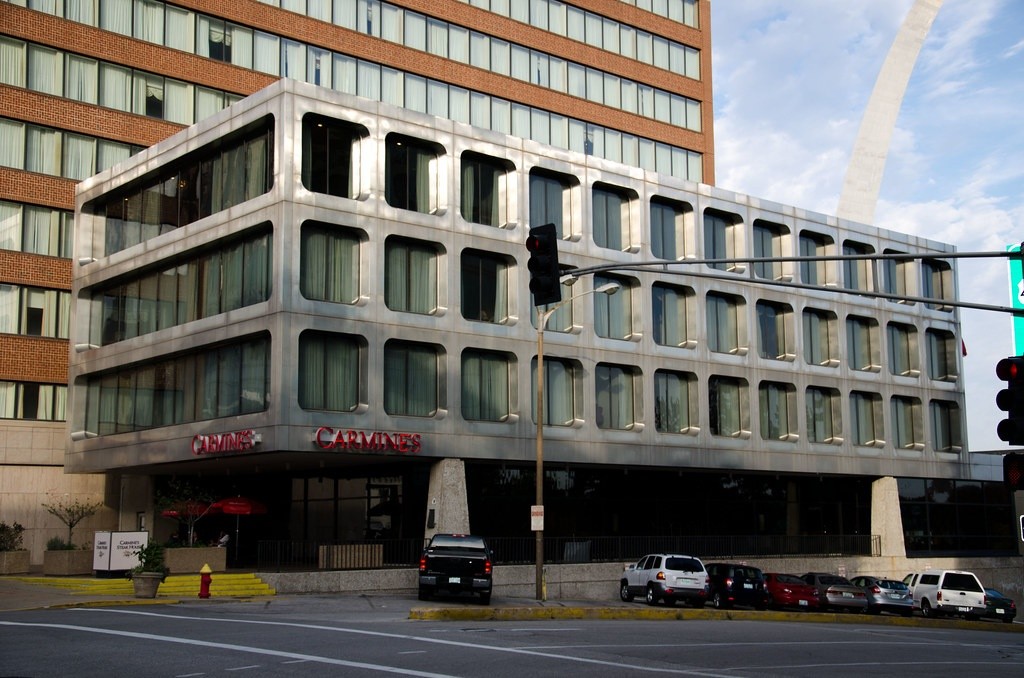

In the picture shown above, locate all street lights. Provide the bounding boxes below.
[535,281,621,602]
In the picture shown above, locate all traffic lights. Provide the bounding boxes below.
[1003,451,1024,491]
[526,223,562,306]
[996,355,1024,445]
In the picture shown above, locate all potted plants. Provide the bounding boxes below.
[0,520,32,574]
[124,536,172,599]
[150,474,227,573]
[39,489,105,575]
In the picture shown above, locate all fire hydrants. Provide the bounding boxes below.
[198,563,213,599]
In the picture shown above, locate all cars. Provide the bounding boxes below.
[963,588,1017,623]
[848,574,916,618]
[799,572,869,613]
[762,573,822,613]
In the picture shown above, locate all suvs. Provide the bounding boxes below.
[897,569,988,621]
[417,534,494,606]
[620,552,711,610]
[687,562,773,611]
